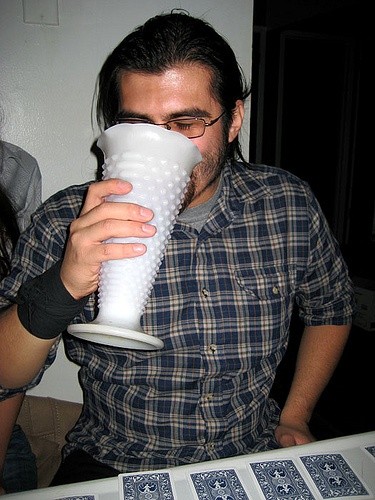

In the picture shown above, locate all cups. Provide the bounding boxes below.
[114,111,227,140]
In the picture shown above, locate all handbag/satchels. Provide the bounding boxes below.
[15,259,90,340]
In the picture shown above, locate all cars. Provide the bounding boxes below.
[68,123,204,351]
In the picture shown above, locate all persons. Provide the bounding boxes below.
[0,13,356,487]
[0,138,43,494]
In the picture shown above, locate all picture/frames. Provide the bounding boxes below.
[1,430,375,500]
